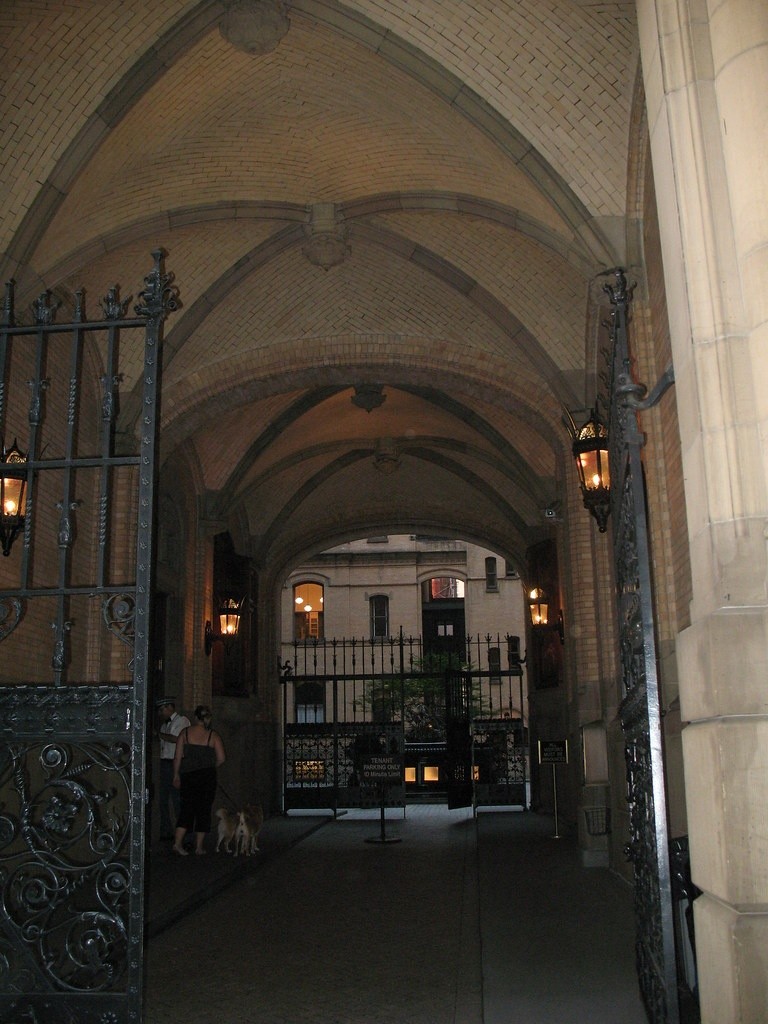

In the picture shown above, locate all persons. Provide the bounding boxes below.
[172,706,225,856]
[145,696,191,841]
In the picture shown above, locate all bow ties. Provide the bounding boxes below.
[165,717,171,724]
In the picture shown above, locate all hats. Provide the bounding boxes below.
[155,696,176,706]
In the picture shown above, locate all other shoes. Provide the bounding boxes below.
[172,845,190,856]
[194,848,206,855]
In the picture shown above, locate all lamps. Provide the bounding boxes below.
[572,407,612,534]
[295,586,312,612]
[204,597,246,656]
[1,438,31,556]
[527,584,564,644]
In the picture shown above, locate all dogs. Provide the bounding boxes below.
[215,803,264,857]
